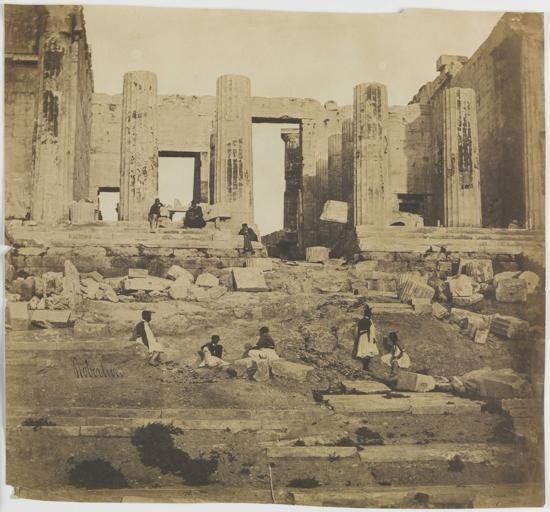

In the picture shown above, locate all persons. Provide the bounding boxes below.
[356,306,379,372]
[135,310,164,366]
[382,331,412,378]
[181,199,206,229]
[196,334,231,369]
[148,198,164,233]
[246,326,280,362]
[239,223,258,255]
